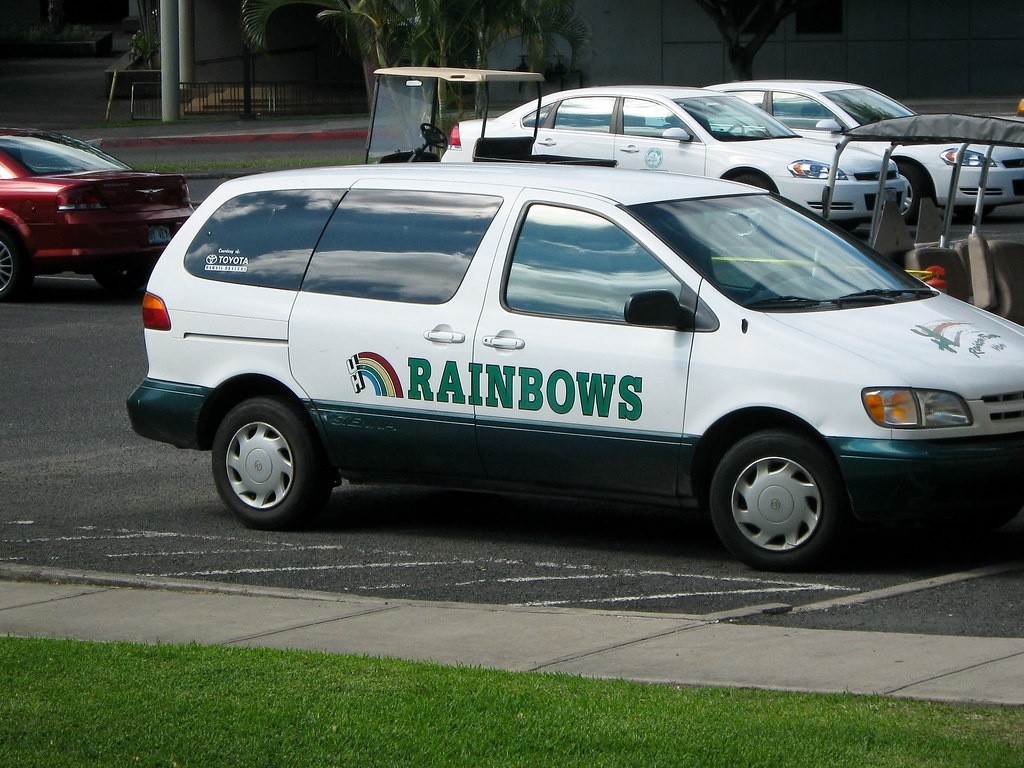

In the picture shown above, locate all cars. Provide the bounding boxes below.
[437,84,909,249]
[0,126,196,302]
[643,78,1023,225]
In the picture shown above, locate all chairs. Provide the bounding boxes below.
[906,232,1024,327]
[473,136,534,163]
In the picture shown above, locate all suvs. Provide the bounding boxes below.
[125,161,1024,575]
